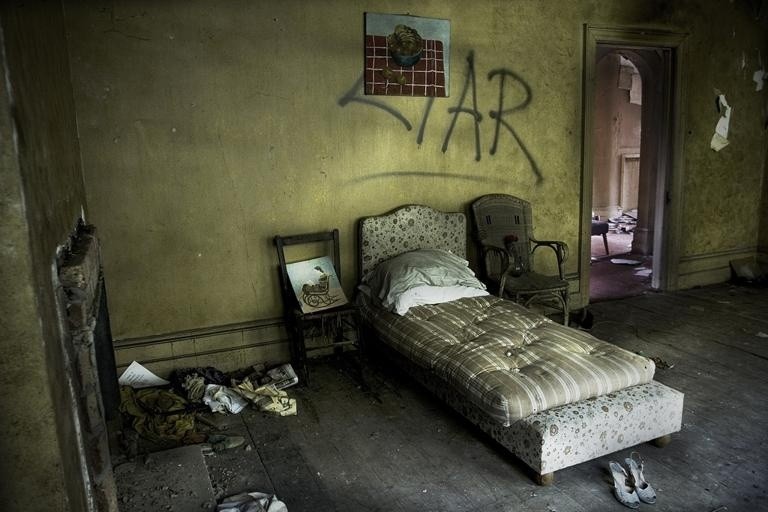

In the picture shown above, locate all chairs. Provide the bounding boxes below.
[472,192,572,327]
[272,228,371,388]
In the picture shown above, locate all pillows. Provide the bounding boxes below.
[359,249,490,320]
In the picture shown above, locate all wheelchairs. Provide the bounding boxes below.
[302,275,333,307]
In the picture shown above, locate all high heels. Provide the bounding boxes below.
[606,451,658,511]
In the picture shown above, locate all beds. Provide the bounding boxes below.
[353,203,685,487]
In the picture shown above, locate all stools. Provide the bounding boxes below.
[591,219,610,255]
